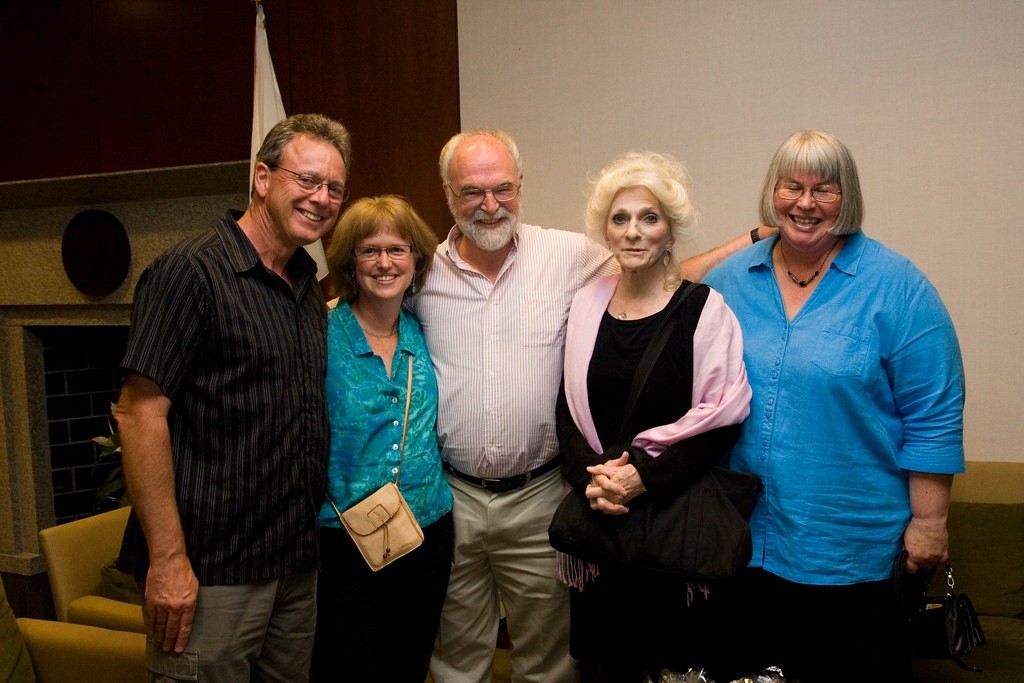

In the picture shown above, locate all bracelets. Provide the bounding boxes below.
[751,227,760,242]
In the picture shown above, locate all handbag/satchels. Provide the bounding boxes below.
[889,550,988,673]
[339,482,425,573]
[548,444,764,577]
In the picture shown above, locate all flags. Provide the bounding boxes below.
[250,12,329,281]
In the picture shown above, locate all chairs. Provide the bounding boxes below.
[8,503,148,683]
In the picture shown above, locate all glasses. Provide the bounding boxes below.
[775,182,842,202]
[273,165,350,203]
[448,181,521,207]
[356,245,413,260]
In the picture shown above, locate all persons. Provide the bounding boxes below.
[309,194,455,683]
[115,114,352,683]
[702,131,966,683]
[403,131,779,683]
[549,152,753,683]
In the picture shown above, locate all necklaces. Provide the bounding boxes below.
[780,239,842,286]
[361,326,399,337]
[619,307,627,320]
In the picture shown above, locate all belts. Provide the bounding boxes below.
[443,454,561,493]
